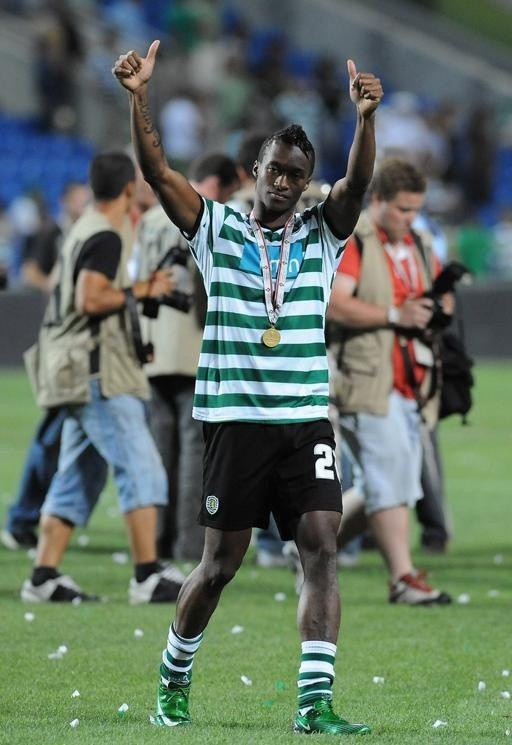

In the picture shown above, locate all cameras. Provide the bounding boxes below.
[418,259,469,339]
[142,245,196,320]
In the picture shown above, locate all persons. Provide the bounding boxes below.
[108,36,388,735]
[2,132,457,612]
[0,0,512,294]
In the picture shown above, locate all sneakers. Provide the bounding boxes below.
[283,541,305,596]
[4,529,37,550]
[157,665,191,727]
[129,565,185,605]
[390,573,449,604]
[293,700,371,735]
[21,574,98,604]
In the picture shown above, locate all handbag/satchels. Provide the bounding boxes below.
[438,336,474,422]
